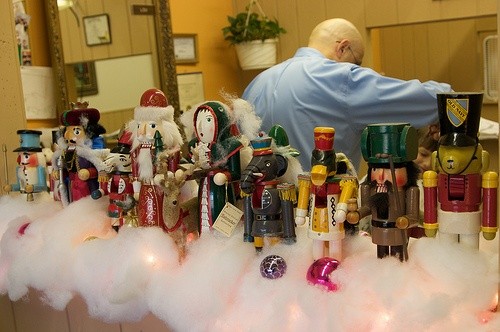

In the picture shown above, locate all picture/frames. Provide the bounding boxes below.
[173,33,200,65]
[82,13,112,47]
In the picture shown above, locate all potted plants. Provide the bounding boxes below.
[219,3,287,71]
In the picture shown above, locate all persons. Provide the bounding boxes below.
[347,122,419,263]
[92,131,141,234]
[5,129,48,201]
[423,92,498,254]
[179,102,244,235]
[410,123,441,174]
[240,18,455,177]
[50,108,106,204]
[295,127,358,261]
[120,88,184,226]
